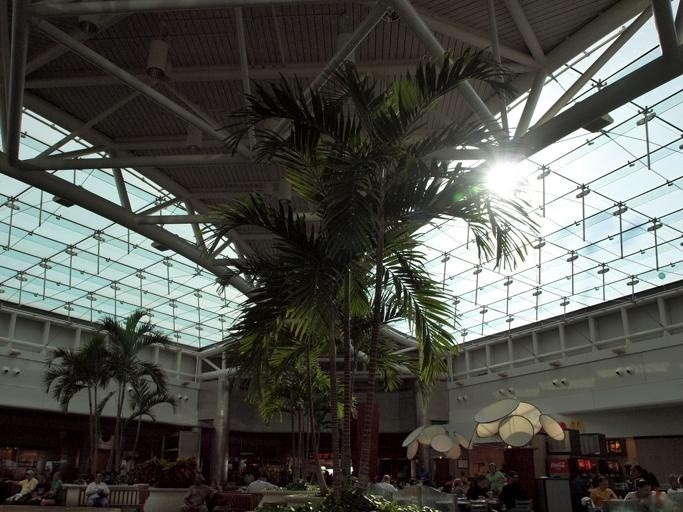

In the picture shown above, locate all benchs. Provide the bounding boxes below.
[0,480,144,512]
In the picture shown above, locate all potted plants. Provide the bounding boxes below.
[141,455,205,512]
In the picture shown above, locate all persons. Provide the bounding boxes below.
[589,464,683,512]
[376,474,397,498]
[499,470,528,512]
[85,473,110,505]
[245,473,278,494]
[183,477,221,511]
[417,462,508,500]
[4,469,65,506]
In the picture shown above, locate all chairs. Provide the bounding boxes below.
[446,492,534,511]
